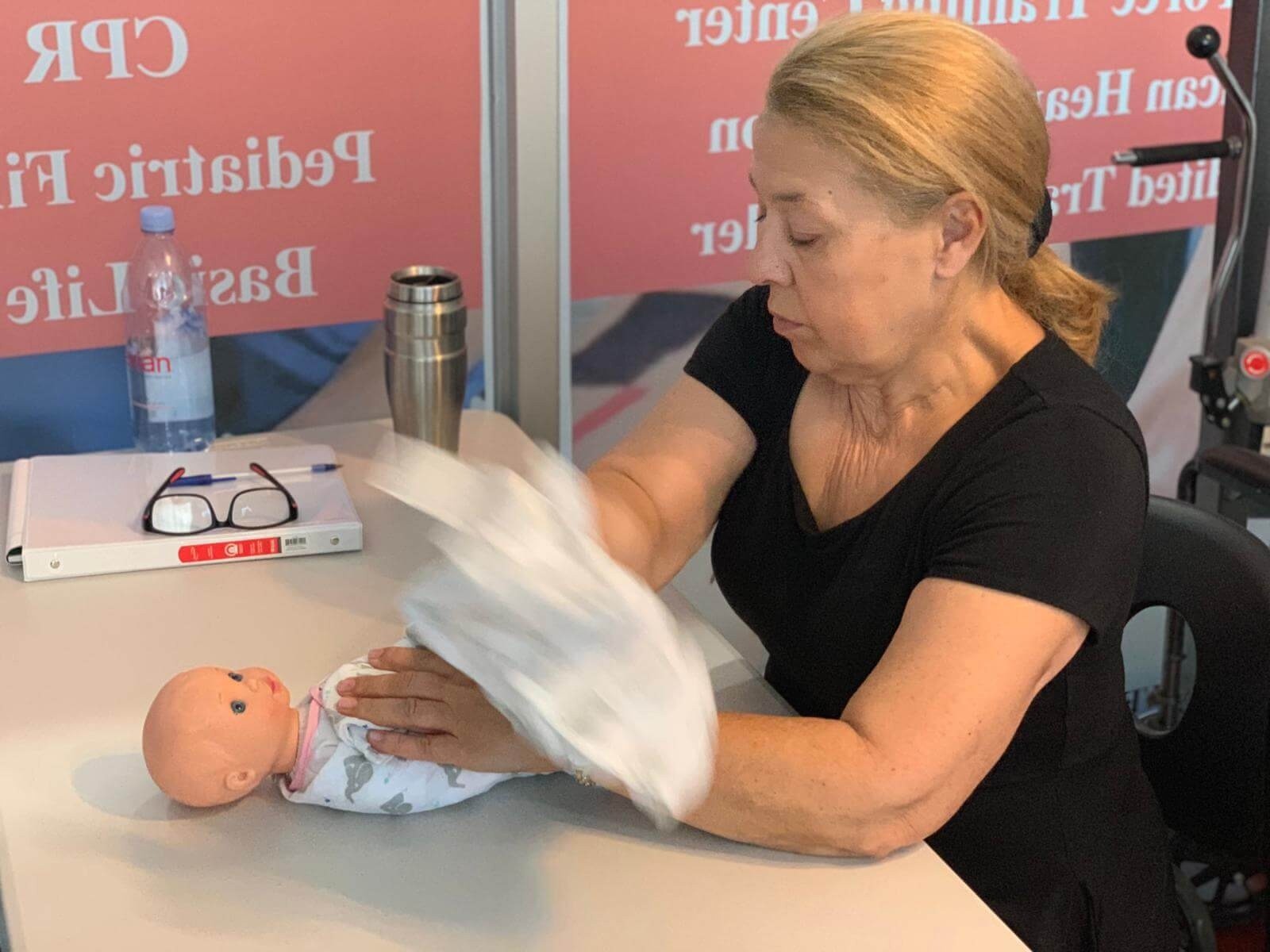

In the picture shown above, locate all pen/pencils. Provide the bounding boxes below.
[172,463,343,485]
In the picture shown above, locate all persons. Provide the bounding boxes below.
[334,10,1220,952]
[141,634,564,817]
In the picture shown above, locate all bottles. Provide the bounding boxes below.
[119,202,216,457]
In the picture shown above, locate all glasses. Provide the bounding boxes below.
[142,462,299,536]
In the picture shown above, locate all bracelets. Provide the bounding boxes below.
[573,768,595,787]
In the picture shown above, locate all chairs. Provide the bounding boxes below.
[1122,495,1269,951]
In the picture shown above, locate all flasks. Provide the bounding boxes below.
[384,267,470,459]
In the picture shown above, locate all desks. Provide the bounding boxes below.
[1,411,1044,951]
